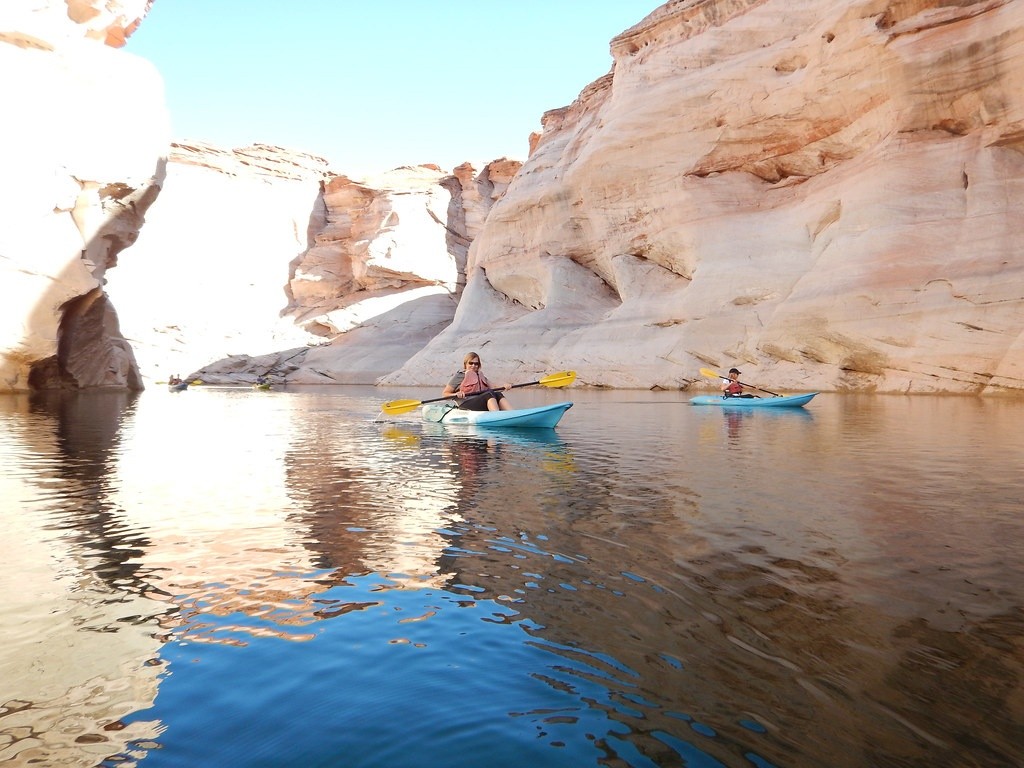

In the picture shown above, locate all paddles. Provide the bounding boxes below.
[155,378,203,386]
[383,428,576,473]
[252,374,272,390]
[381,370,575,415]
[700,366,789,397]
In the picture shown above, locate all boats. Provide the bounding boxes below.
[421,402,573,429]
[254,383,270,388]
[191,380,204,385]
[688,392,821,407]
[170,383,187,390]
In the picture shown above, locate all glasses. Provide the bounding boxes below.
[467,361,479,365]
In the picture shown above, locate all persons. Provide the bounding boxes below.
[254,375,269,385]
[442,352,512,412]
[720,368,762,400]
[168,374,183,384]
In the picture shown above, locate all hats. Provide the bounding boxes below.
[729,368,741,374]
